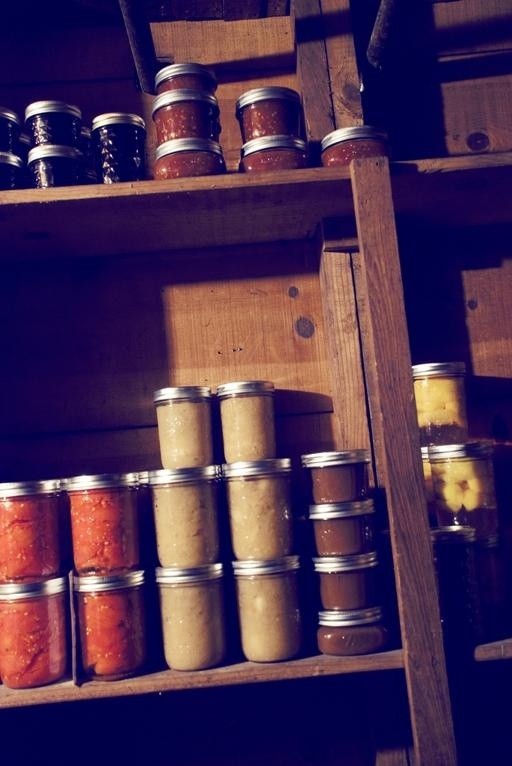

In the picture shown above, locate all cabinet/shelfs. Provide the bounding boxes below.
[3,2,512,764]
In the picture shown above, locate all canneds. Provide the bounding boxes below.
[410,363,499,646]
[0,382,386,688]
[0,62,384,193]
